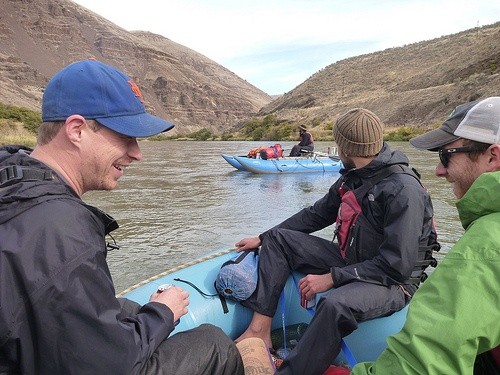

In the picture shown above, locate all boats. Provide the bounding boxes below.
[233,155,344,173]
[220,153,248,171]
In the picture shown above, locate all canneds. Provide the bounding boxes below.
[300,292,316,309]
[157,284,180,326]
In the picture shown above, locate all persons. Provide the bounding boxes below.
[289,125,314,157]
[349,96,500,375]
[0,58,245,375]
[233,108,434,375]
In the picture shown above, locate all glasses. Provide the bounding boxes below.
[439,144,491,168]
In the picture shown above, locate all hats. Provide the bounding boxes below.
[42,58,176,138]
[409,96,500,149]
[334,108,383,157]
[297,125,307,131]
